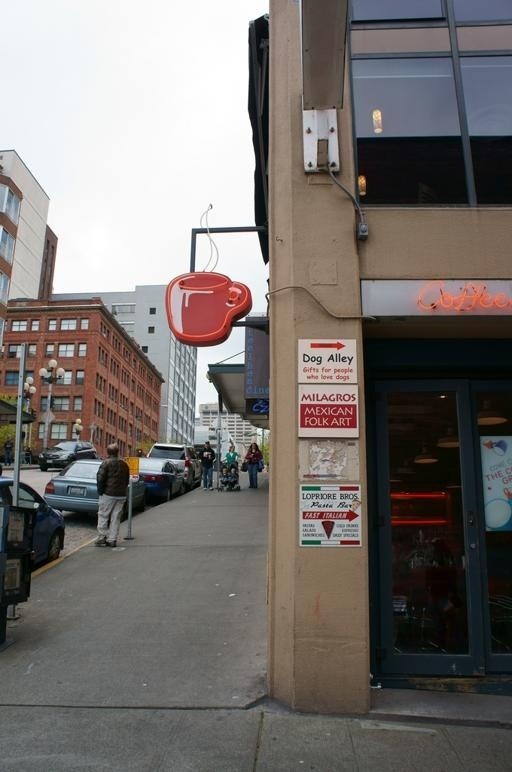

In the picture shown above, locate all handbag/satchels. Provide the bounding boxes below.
[241,462,247,472]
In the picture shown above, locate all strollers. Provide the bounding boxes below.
[218,461,241,492]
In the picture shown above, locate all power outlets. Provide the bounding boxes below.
[357,221,370,241]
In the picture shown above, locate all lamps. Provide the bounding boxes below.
[297,0,369,241]
[371,105,383,134]
[388,398,509,485]
[357,171,366,197]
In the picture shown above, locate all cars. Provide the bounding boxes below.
[134,457,186,502]
[0,477,65,574]
[43,458,148,523]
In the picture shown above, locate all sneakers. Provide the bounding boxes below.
[106,541,116,547]
[95,540,106,545]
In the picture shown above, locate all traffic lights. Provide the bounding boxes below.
[138,449,142,457]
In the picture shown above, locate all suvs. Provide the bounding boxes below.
[39,439,99,472]
[142,441,201,490]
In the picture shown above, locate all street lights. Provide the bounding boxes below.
[23,375,38,451]
[37,357,67,450]
[74,418,84,442]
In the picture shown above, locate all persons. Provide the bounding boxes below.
[228,467,238,485]
[25,446,36,463]
[223,446,238,466]
[198,441,215,490]
[221,467,229,484]
[94,443,130,547]
[245,443,264,489]
[4,437,14,466]
[137,449,145,457]
